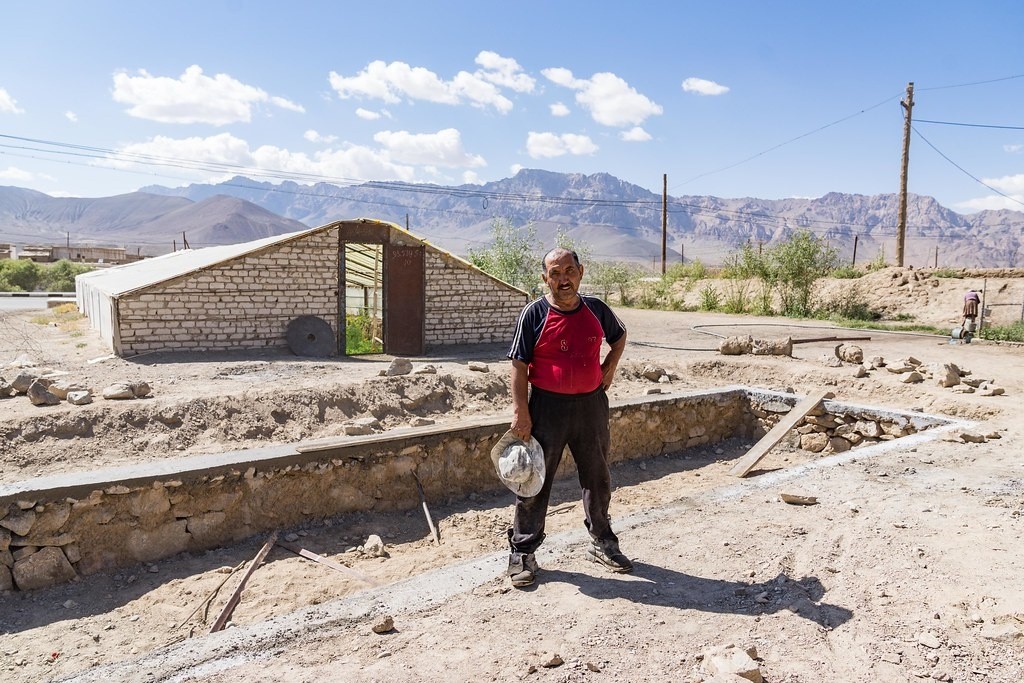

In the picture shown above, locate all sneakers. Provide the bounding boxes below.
[507,552,539,586]
[585,539,634,571]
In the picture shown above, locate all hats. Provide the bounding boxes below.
[491,428,545,497]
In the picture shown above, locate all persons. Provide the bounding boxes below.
[963,290,980,322]
[508,249,632,588]
